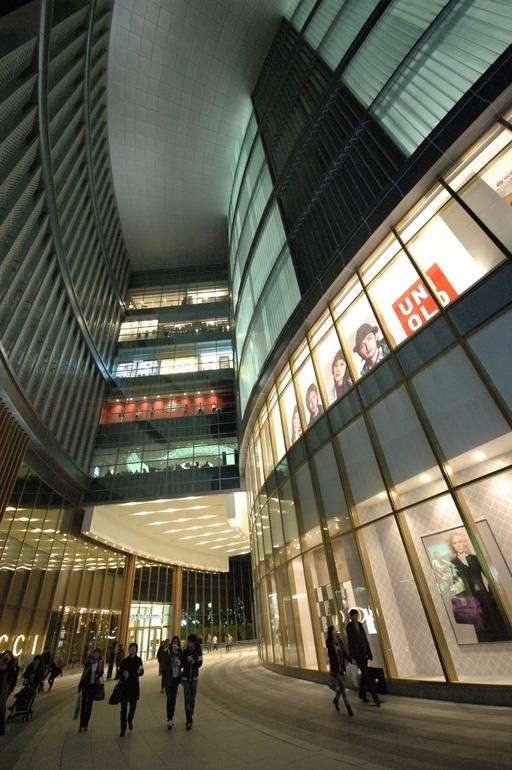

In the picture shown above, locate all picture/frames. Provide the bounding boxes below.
[420,519,512,645]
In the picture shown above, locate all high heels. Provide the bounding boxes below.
[346,703,353,715]
[332,696,339,710]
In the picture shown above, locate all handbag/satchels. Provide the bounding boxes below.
[92,683,106,701]
[108,681,121,704]
[327,673,342,691]
[451,596,483,625]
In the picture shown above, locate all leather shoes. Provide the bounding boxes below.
[120,721,132,736]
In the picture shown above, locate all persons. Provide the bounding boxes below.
[326,348,353,406]
[305,383,325,429]
[325,625,356,717]
[449,531,512,643]
[0,645,62,737]
[76,633,233,736]
[290,405,303,444]
[345,608,384,705]
[353,323,389,379]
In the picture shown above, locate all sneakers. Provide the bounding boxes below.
[78,727,88,732]
[359,694,379,706]
[167,717,192,730]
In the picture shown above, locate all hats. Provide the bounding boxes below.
[332,349,346,361]
[352,322,378,353]
